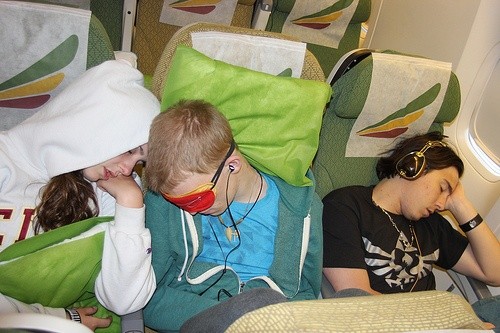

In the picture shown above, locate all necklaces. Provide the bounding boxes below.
[217,164,263,241]
[372,196,414,248]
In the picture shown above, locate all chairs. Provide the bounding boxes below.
[0,0,500,333]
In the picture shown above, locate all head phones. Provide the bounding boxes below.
[395,139,458,180]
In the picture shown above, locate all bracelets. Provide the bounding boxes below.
[459,213,483,232]
[65,308,81,323]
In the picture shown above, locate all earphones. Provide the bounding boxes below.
[229,164,235,172]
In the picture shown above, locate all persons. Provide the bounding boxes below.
[322,133,500,296]
[145,100,374,333]
[0,60,161,333]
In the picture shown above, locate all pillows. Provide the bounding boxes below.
[0,216,121,333]
[161,43,335,185]
